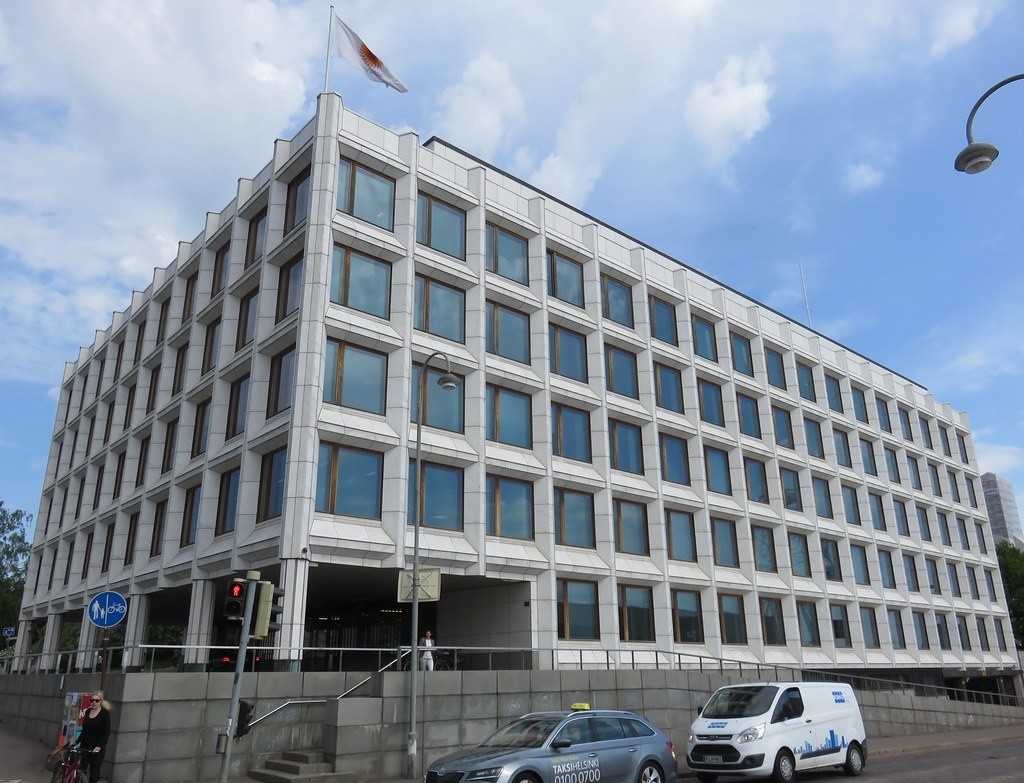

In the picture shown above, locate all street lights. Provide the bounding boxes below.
[407,351,463,781]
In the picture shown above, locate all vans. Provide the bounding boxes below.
[685,680,868,783]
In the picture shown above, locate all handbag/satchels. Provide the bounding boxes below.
[46,742,75,771]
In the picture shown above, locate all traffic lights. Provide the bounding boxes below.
[254,583,286,638]
[223,579,249,619]
[236,701,254,736]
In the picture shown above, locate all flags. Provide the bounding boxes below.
[333,9,411,93]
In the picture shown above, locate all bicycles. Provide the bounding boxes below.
[51,745,97,783]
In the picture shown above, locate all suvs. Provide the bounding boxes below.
[424,702,679,783]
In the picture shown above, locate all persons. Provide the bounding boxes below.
[418,631,435,671]
[171,648,180,668]
[67,689,111,783]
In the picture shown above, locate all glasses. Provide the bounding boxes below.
[90,698,101,703]
